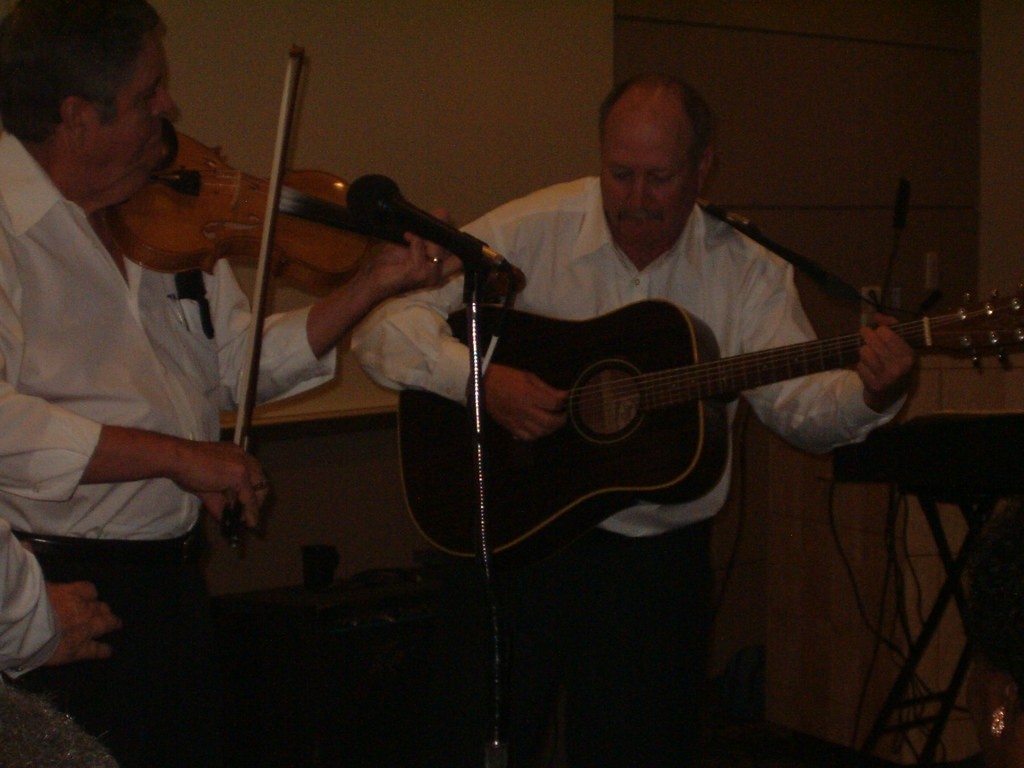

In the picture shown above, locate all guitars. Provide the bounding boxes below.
[399,278,1024,558]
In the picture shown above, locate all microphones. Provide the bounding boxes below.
[346,173,509,273]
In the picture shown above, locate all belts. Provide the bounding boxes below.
[19,533,195,559]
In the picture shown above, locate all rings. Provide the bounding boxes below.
[428,257,442,264]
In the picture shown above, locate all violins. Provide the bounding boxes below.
[97,130,511,286]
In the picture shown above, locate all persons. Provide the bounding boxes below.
[0,516,123,679]
[0,1,462,768]
[349,72,917,768]
